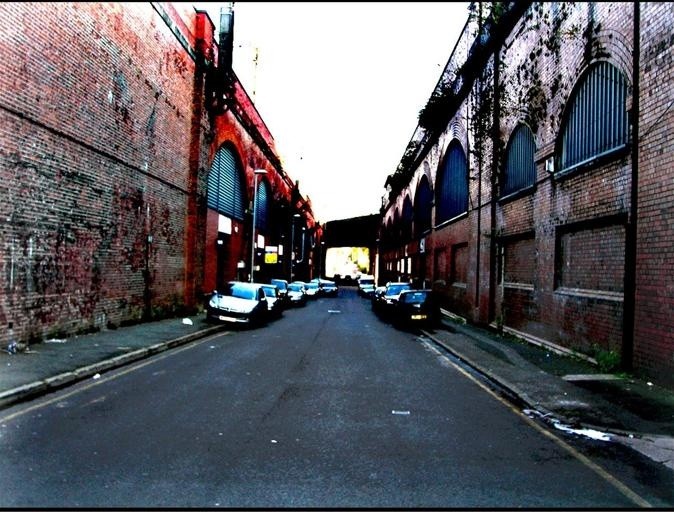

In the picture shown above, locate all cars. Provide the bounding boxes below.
[356,272,442,332]
[206,276,338,329]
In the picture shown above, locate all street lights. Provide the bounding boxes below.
[249,167,270,285]
